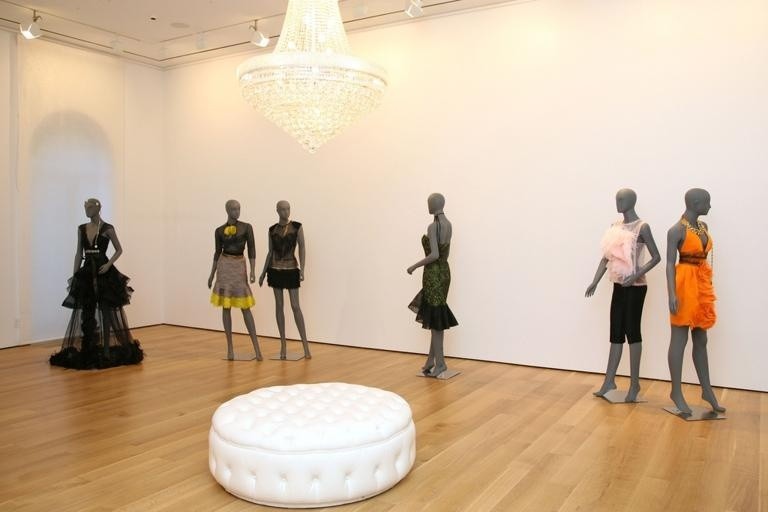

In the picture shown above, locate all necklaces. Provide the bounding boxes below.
[682,215,705,235]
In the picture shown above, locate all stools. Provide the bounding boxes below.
[207,380,418,508]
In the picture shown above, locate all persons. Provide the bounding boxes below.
[49,198,144,369]
[208,200,263,362]
[585,189,661,402]
[666,188,726,413]
[406,193,457,377]
[258,201,311,359]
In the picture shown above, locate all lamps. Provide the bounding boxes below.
[402,0,428,21]
[18,4,45,41]
[244,16,272,52]
[233,0,391,155]
